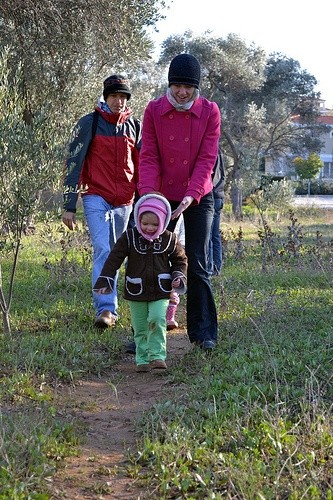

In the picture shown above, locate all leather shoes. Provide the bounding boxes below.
[93,310,117,328]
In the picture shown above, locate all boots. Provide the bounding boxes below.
[167,304,178,329]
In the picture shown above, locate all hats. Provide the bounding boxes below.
[138,198,168,240]
[104,74,131,103]
[168,53,202,90]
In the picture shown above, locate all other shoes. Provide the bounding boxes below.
[199,339,215,351]
[126,342,136,354]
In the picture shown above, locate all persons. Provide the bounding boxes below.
[138,53,226,351]
[62,74,146,326]
[93,194,187,372]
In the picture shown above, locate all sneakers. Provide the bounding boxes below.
[151,360,167,369]
[136,364,151,372]
